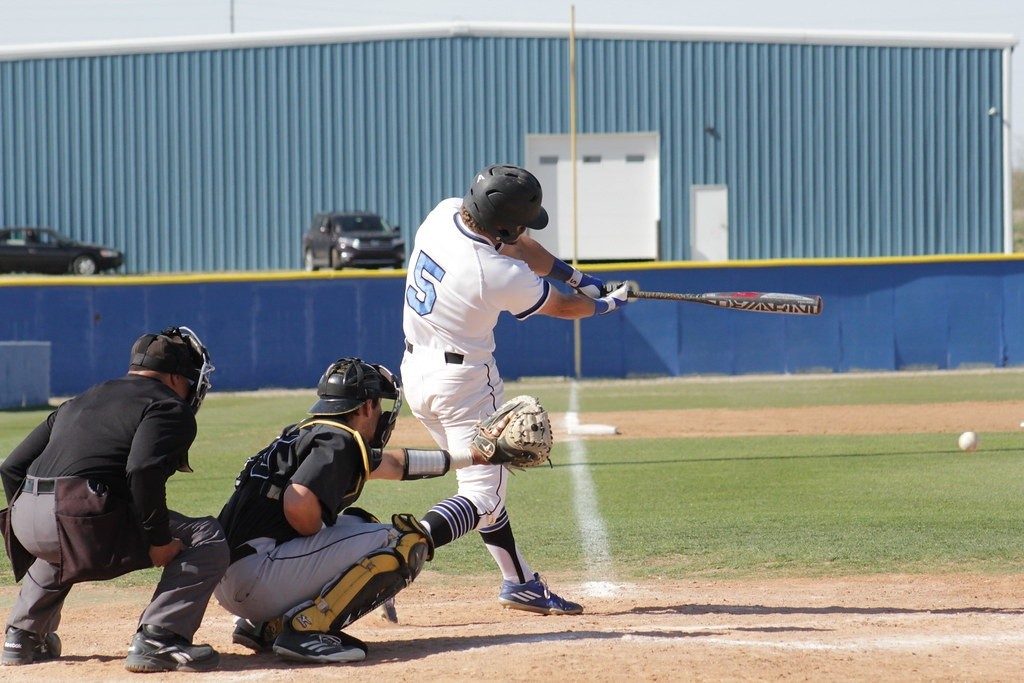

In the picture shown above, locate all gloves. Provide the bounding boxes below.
[593,280,630,315]
[575,274,604,297]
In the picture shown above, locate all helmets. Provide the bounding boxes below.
[463,164,548,245]
[307,358,383,416]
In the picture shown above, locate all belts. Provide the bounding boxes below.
[21,478,55,494]
[228,544,256,564]
[408,343,463,364]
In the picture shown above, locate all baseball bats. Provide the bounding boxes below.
[628,289,824,316]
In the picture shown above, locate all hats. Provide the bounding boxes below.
[131,332,197,371]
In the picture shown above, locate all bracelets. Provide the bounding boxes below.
[450,449,474,470]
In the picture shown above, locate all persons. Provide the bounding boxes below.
[0,326,231,673]
[213,357,554,662]
[375,164,629,623]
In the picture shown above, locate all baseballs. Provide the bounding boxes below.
[959,432,979,452]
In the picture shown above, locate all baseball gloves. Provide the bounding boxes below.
[470,394,553,467]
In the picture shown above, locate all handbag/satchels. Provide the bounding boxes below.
[0,479,38,584]
[54,478,141,584]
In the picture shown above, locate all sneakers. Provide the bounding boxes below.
[232,625,269,652]
[1,627,61,665]
[273,628,365,662]
[500,573,583,615]
[125,625,219,672]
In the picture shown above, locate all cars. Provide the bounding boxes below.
[0,226,123,277]
[302,211,405,271]
